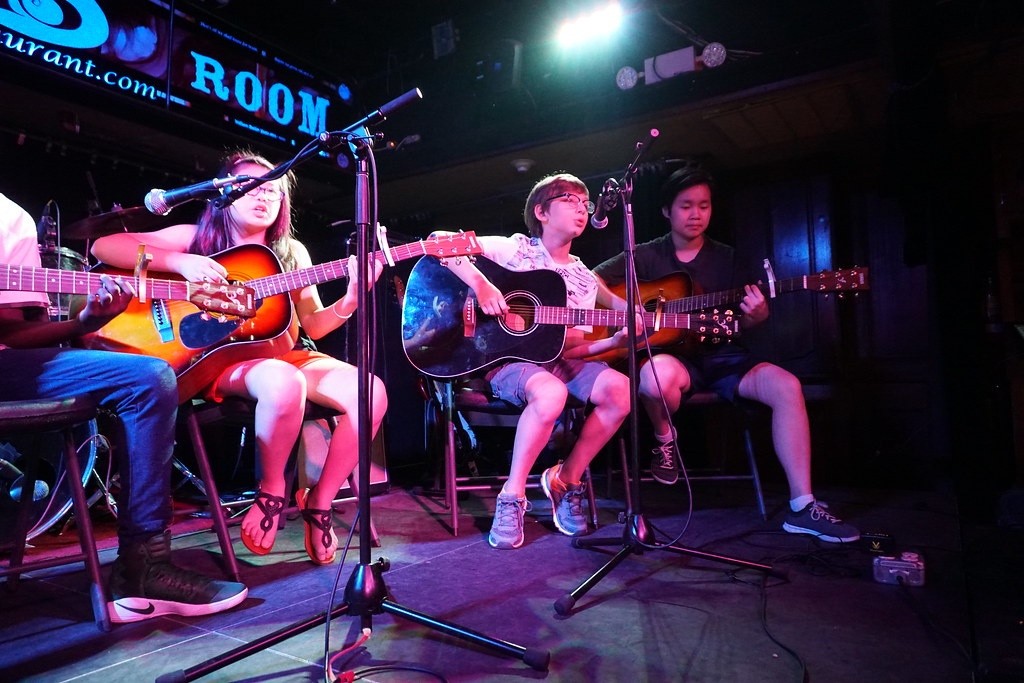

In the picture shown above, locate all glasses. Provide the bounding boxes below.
[234,181,285,202]
[538,192,596,215]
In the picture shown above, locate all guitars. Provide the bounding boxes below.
[583,261,872,369]
[398,250,743,383]
[0,262,260,327]
[71,225,484,406]
[388,272,479,468]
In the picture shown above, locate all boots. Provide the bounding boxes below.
[105,529,249,623]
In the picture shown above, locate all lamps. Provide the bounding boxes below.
[616,42,727,91]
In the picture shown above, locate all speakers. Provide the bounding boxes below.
[286,412,390,506]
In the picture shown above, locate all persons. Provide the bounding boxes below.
[89,154,385,566]
[0,190,249,625]
[427,173,646,549]
[587,168,861,543]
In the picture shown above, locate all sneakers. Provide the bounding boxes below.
[488,483,533,550]
[540,460,588,536]
[650,427,679,485]
[782,499,860,543]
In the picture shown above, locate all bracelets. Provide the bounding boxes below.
[333,301,353,319]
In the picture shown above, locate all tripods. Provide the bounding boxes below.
[153,86,551,683]
[553,130,799,615]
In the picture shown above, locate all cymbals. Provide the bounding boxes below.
[58,205,166,240]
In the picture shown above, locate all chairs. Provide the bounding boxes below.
[606,389,770,522]
[0,393,112,632]
[174,396,380,584]
[394,275,598,536]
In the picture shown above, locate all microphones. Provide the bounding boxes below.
[9,474,49,502]
[145,175,251,216]
[34,202,52,243]
[590,181,612,228]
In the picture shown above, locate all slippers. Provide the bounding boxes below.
[295,488,336,565]
[241,481,286,555]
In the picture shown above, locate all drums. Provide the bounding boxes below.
[0,413,100,552]
[37,243,91,320]
